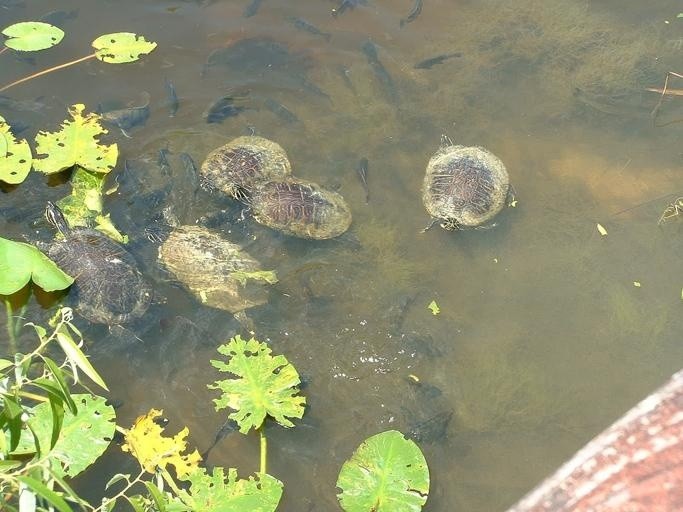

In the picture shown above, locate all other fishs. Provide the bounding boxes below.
[0,1,388,468]
